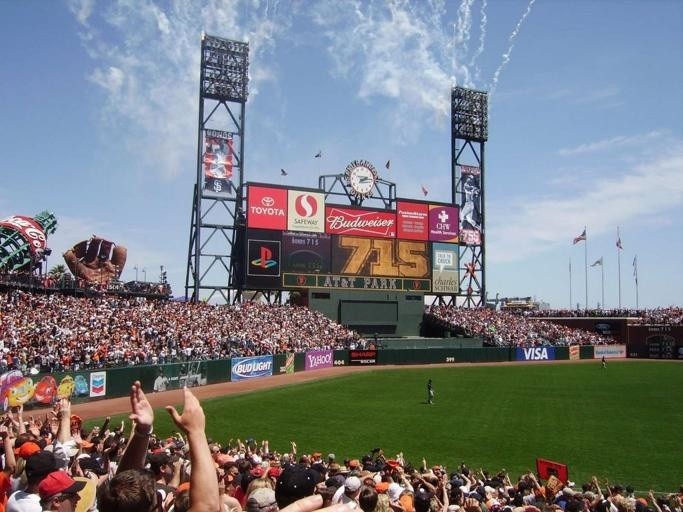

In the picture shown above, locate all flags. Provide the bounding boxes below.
[571,228,586,245]
[614,231,624,250]
[421,186,428,196]
[590,256,603,267]
[384,159,390,169]
[314,150,321,159]
[631,256,637,285]
[279,167,288,176]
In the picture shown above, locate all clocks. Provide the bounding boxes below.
[349,165,374,194]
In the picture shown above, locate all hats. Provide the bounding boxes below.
[326,474,345,487]
[76,440,94,449]
[276,465,321,501]
[248,487,277,508]
[150,452,179,471]
[634,498,649,508]
[38,471,87,505]
[0,472,13,494]
[25,449,66,480]
[313,452,360,468]
[214,443,279,477]
[73,457,108,476]
[416,493,432,507]
[399,489,414,512]
[386,482,406,502]
[19,438,47,458]
[345,476,361,491]
[375,482,389,493]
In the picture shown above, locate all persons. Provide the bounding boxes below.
[425,298,683,349]
[93,380,222,512]
[314,449,681,512]
[1,395,314,512]
[600,355,606,369]
[425,378,434,405]
[0,265,373,380]
[459,173,480,231]
